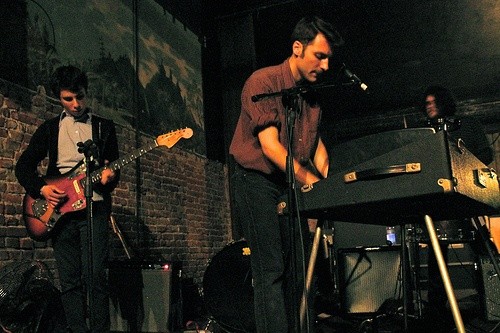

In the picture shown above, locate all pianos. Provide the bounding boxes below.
[276,119,500,333]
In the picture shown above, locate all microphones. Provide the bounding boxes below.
[342,66,368,91]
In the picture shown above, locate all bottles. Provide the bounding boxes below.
[386,225,397,245]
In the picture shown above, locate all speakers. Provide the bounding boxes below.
[85,265,172,333]
[338,242,408,313]
[478,253,500,321]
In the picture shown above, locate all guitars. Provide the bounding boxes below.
[22,124,194,242]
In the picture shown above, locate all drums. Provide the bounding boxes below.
[309,231,330,274]
[201,239,258,333]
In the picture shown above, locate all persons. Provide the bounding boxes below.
[229,17,343,333]
[15,65,120,333]
[425,86,495,165]
